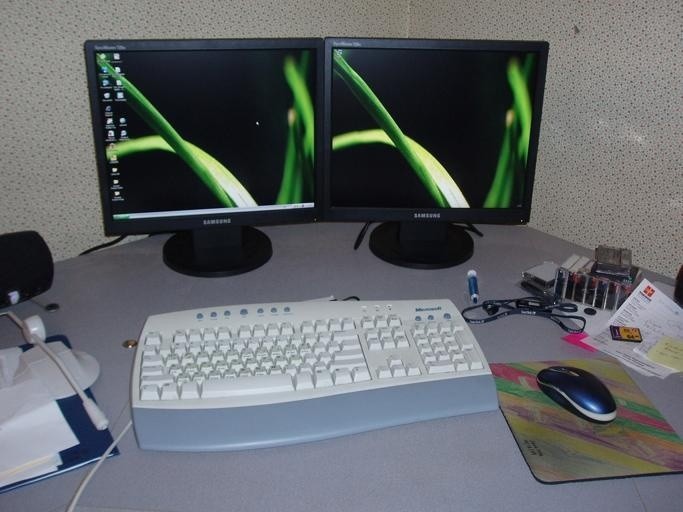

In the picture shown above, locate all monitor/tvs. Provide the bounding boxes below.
[324,34,550,270]
[84,37,323,279]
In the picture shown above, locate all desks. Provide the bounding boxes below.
[0,222,682,512]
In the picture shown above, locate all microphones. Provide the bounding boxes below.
[29,333,110,430]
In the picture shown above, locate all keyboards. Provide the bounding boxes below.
[130,299,498,451]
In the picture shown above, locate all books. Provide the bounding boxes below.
[0,331,118,493]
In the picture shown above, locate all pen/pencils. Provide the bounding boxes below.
[467,269,479,304]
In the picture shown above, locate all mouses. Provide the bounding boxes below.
[536,366,617,423]
